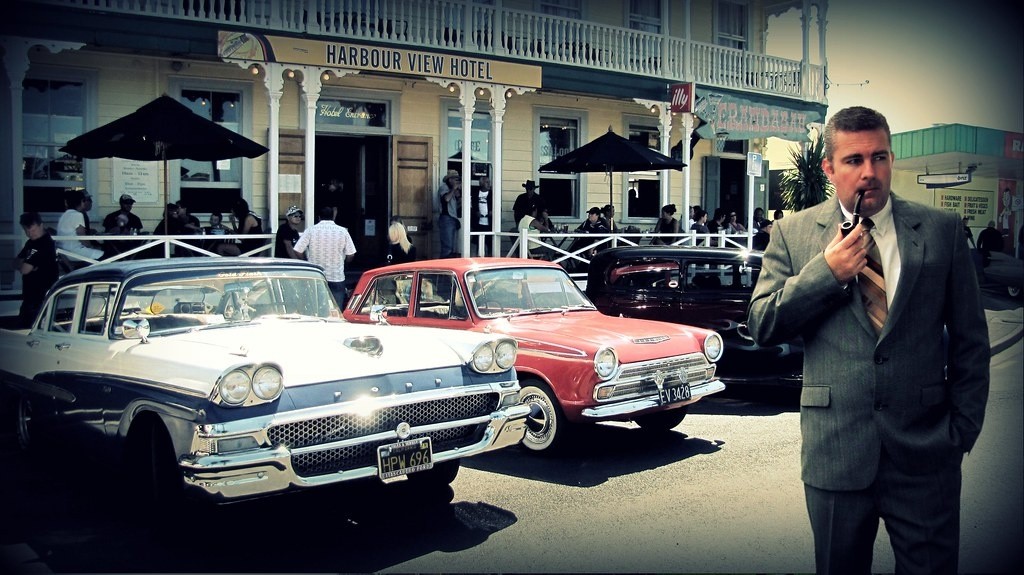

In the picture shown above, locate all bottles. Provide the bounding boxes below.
[130,228,137,235]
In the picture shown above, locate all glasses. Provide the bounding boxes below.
[173,211,179,214]
[290,216,302,218]
[729,213,736,217]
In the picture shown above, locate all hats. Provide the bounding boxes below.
[119,194,135,203]
[443,170,460,182]
[522,180,539,188]
[82,190,92,197]
[586,207,600,214]
[286,205,304,216]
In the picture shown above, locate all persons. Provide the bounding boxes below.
[962,218,1003,252]
[274,183,416,313]
[746,106,990,575]
[652,204,783,250]
[101,194,144,261]
[471,177,503,257]
[152,194,264,257]
[512,170,549,257]
[14,211,57,318]
[570,205,619,256]
[58,189,105,262]
[437,170,461,258]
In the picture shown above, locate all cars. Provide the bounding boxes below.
[970,248,1023,299]
[0,254,532,539]
[344,256,726,459]
[586,245,806,387]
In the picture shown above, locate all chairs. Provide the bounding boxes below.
[47,227,80,275]
[509,227,546,259]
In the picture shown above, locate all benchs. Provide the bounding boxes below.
[52,312,225,335]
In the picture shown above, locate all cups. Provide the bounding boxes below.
[564,226,568,232]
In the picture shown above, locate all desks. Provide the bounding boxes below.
[93,231,150,260]
[541,229,581,270]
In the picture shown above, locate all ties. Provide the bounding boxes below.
[858,219,889,339]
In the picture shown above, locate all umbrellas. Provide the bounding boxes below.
[538,124,688,233]
[58,91,271,234]
